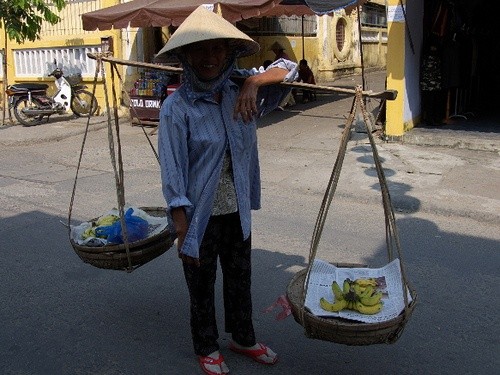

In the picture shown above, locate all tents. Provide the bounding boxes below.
[82,0,314,60]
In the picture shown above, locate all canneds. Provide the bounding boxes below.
[130,78,160,95]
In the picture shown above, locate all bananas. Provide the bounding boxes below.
[320,278,383,314]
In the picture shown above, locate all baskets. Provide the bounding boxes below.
[67,73,83,86]
[69,207,174,274]
[286,262,415,346]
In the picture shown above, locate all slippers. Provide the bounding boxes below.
[199,350,229,375]
[230,338,277,365]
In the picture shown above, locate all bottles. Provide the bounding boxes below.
[127,56,180,109]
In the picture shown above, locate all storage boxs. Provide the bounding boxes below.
[167,83,181,95]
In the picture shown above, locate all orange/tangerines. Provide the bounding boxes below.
[82,214,120,240]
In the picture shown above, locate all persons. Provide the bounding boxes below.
[270,41,316,110]
[155,6,299,375]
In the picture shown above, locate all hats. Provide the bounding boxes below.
[268,42,286,50]
[154,7,259,64]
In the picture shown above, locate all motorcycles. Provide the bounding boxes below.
[5,58,98,127]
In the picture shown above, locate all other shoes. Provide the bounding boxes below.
[277,106,285,111]
